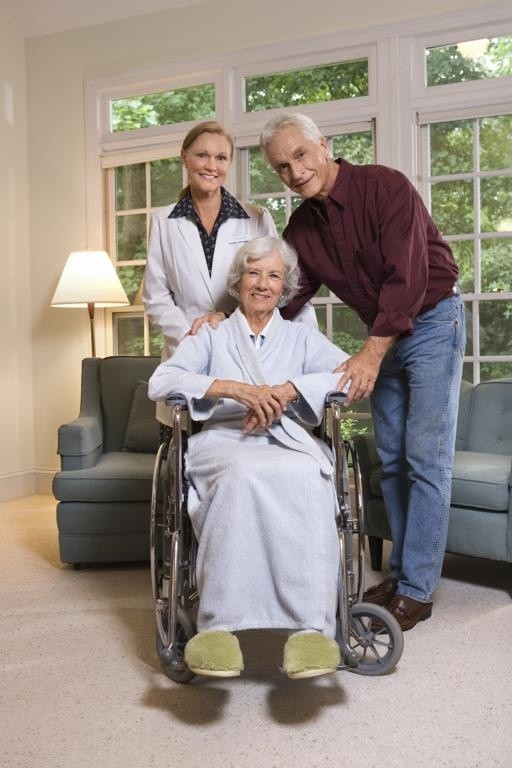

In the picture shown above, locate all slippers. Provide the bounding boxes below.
[281,632,341,680]
[181,629,246,678]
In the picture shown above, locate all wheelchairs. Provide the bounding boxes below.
[147,390,404,685]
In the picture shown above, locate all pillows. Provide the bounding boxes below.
[122,380,160,454]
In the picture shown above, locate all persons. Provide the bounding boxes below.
[144,236,367,678]
[144,122,322,364]
[257,117,465,633]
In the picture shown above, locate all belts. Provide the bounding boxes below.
[440,286,459,301]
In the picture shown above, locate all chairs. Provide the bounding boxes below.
[52,356,168,571]
[351,378,512,571]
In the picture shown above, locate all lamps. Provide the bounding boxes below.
[50,250,131,357]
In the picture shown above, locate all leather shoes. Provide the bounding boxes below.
[368,593,433,635]
[362,577,399,607]
